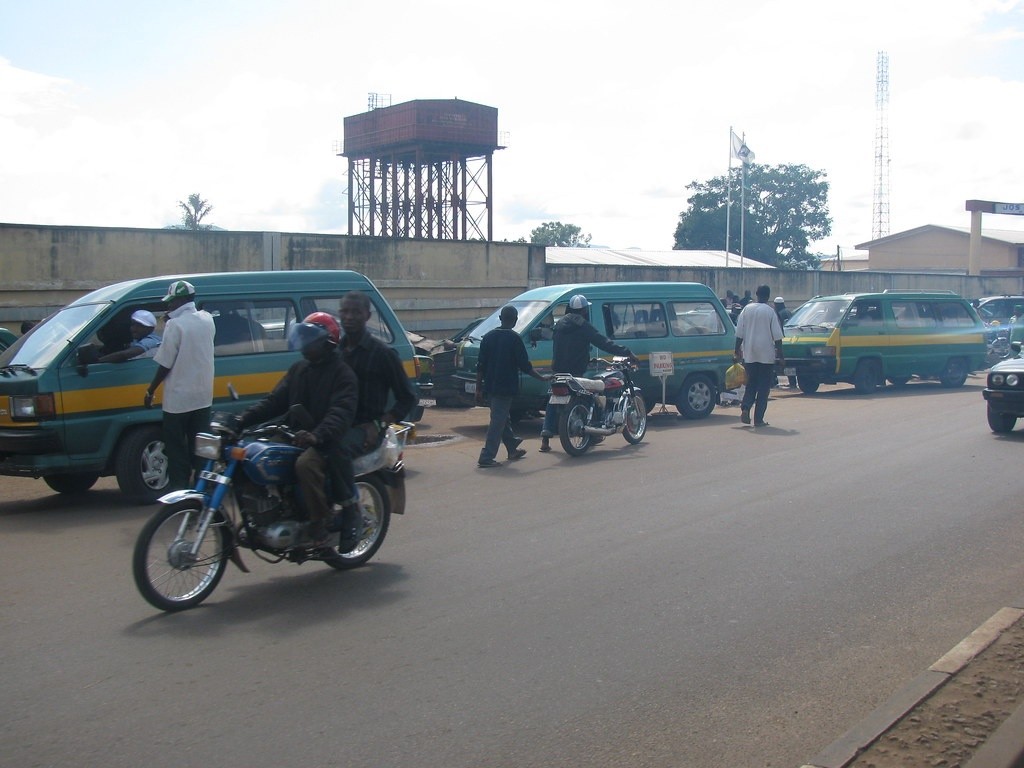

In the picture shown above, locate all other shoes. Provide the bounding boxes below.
[741,407,751,424]
[338,504,363,553]
[477,459,501,466]
[755,421,769,427]
[539,440,552,451]
[311,508,330,540]
[508,448,527,460]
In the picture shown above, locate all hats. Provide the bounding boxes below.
[774,296,784,303]
[570,294,593,310]
[131,310,157,327]
[162,280,195,304]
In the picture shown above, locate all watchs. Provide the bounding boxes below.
[145,389,154,398]
[376,417,390,431]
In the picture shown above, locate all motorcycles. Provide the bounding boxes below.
[132,383,416,611]
[984,322,1011,359]
[546,354,647,457]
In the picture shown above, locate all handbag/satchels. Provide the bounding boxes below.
[725,353,746,390]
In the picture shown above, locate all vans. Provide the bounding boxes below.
[450,280,736,421]
[774,288,986,395]
[1,267,423,504]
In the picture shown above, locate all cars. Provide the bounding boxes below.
[971,294,1024,339]
[250,308,742,393]
[981,340,1024,432]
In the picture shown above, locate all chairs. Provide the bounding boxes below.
[649,308,665,325]
[634,309,648,327]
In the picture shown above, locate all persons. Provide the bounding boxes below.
[719,288,797,392]
[475,305,554,468]
[20,320,36,335]
[95,309,162,364]
[1007,304,1024,358]
[733,284,783,427]
[232,311,360,549]
[317,290,417,555]
[539,294,638,452]
[142,279,217,496]
[972,298,993,328]
[845,303,875,322]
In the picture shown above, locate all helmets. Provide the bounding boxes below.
[296,312,340,348]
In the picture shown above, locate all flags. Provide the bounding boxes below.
[730,130,756,166]
